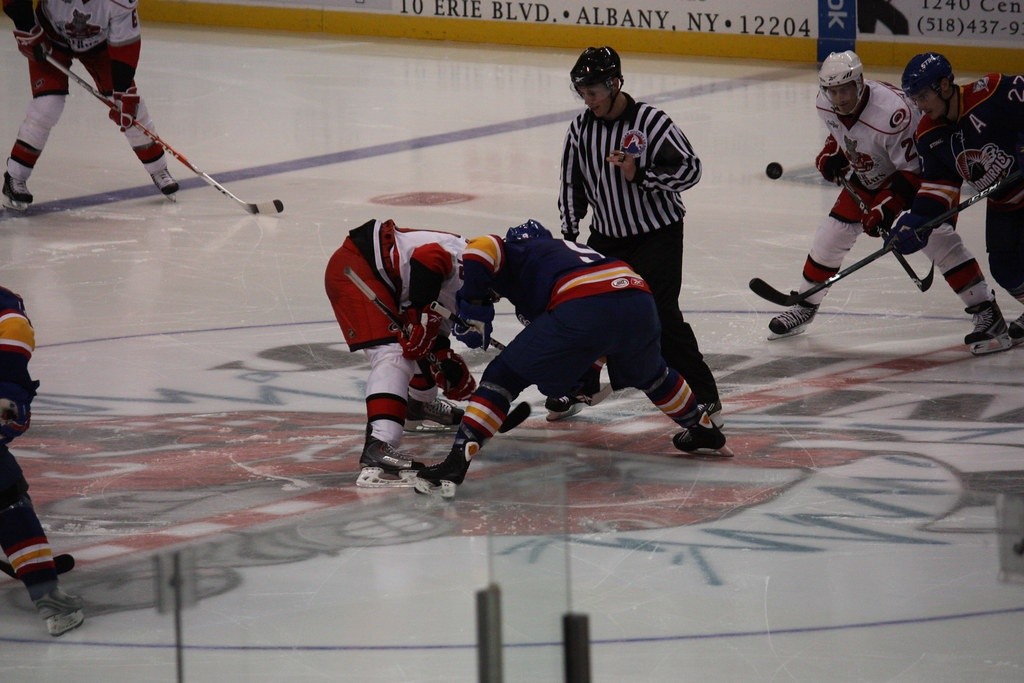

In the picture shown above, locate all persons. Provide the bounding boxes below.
[324,222,475,474]
[883,52,1024,345]
[2,0,178,202]
[0,288,83,623]
[544,46,724,420]
[768,50,1012,345]
[414,221,734,499]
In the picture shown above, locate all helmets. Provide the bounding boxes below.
[902,52,955,97]
[570,46,622,87]
[506,219,553,240]
[818,50,864,101]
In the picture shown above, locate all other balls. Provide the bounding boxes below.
[766,162,784,180]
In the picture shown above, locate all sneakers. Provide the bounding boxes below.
[703,401,725,429]
[2,171,34,211]
[150,167,180,202]
[1008,313,1024,345]
[27,580,84,637]
[405,396,464,433]
[545,372,601,421]
[767,301,820,340]
[417,424,488,498]
[961,289,1013,356]
[672,426,734,457]
[355,423,425,489]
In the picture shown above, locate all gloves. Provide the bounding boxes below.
[429,349,477,402]
[814,136,849,186]
[399,306,443,358]
[451,314,494,351]
[0,400,32,446]
[887,210,934,255]
[12,26,52,65]
[861,189,903,238]
[108,87,140,133]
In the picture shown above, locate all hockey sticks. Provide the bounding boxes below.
[429,299,613,407]
[0,553,78,581]
[44,54,285,217]
[748,167,1023,309]
[344,266,531,434]
[835,172,935,294]
